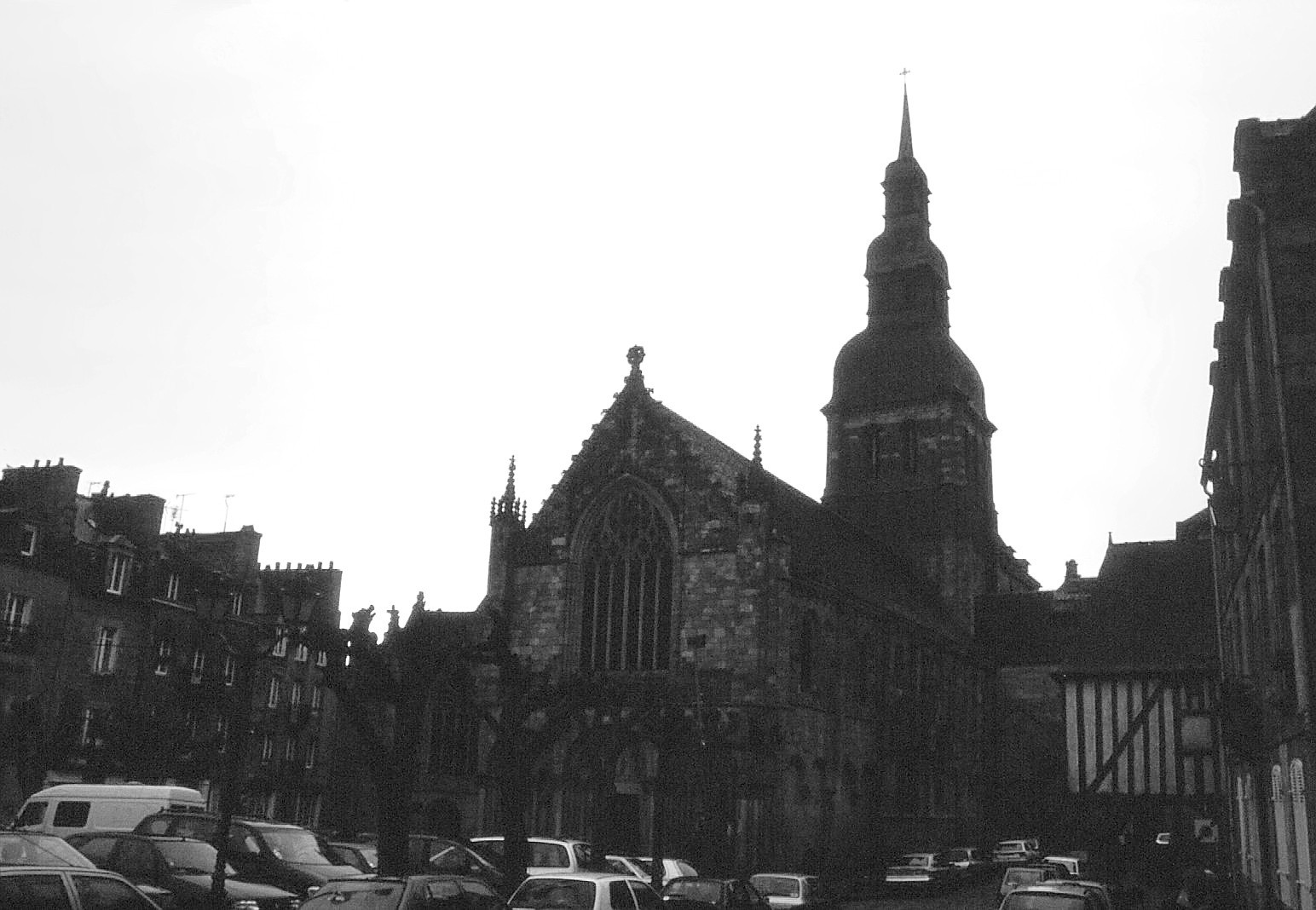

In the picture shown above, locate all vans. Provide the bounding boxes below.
[10,785,205,837]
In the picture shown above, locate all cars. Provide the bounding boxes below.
[0,783,1112,910]
